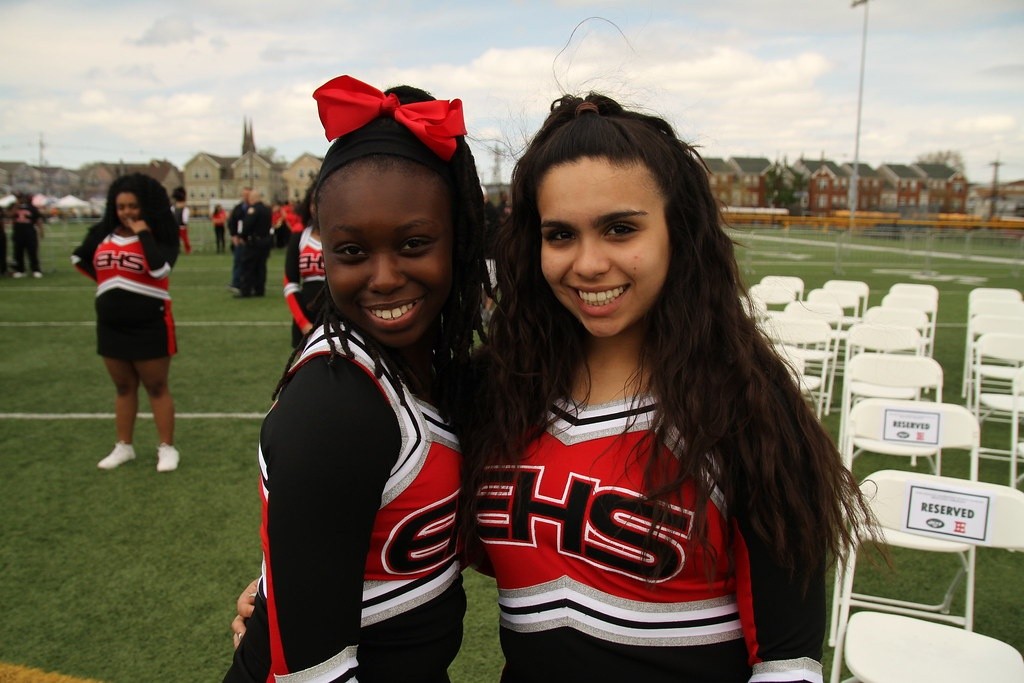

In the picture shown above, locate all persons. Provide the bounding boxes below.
[1,185,45,280]
[224,75,496,683]
[169,183,335,352]
[70,171,181,473]
[230,92,827,683]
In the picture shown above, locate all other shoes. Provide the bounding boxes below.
[232,292,265,298]
[33,272,42,278]
[13,272,26,278]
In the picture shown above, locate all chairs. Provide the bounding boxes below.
[730,275,1024,683]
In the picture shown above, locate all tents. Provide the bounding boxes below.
[0,192,106,219]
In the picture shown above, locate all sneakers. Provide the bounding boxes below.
[156,443,181,472]
[96,442,136,470]
